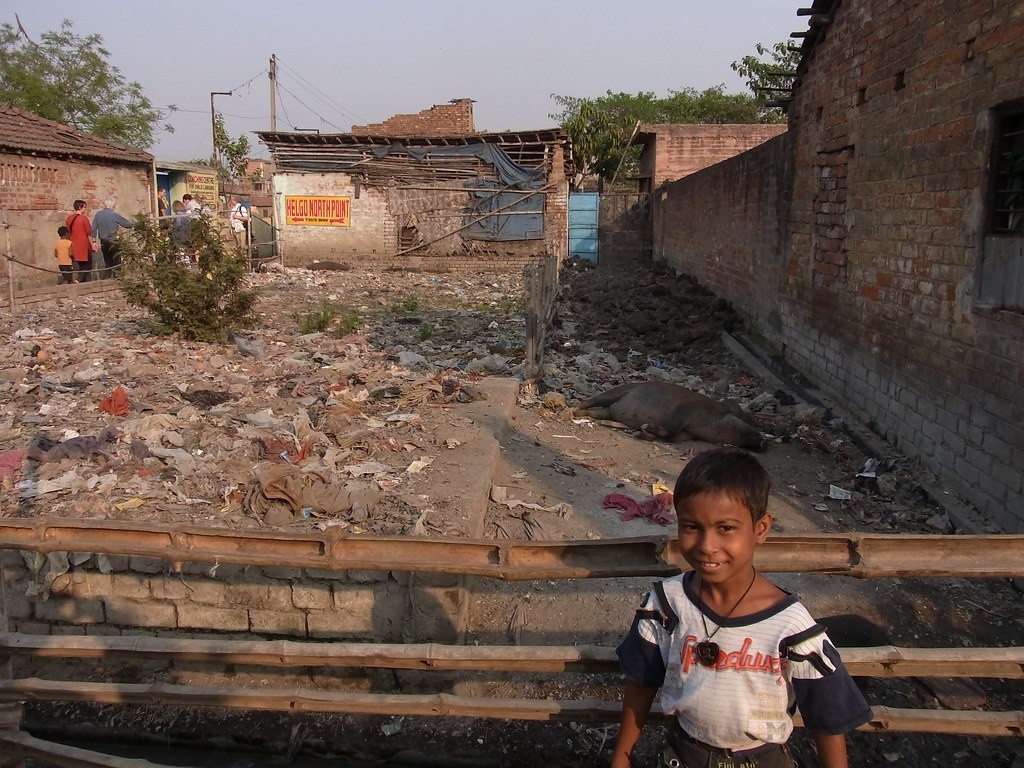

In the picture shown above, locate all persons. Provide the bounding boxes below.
[55,183,213,284]
[229,196,252,261]
[610,449,875,768]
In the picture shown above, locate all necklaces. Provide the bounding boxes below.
[697,564,756,666]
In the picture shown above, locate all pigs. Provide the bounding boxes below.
[572,381,769,454]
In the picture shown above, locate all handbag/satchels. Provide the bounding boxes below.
[174,248,191,269]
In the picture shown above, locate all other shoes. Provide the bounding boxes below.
[75,279,80,283]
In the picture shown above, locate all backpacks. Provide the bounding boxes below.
[239,201,252,230]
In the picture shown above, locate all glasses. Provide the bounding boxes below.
[83,206,86,208]
